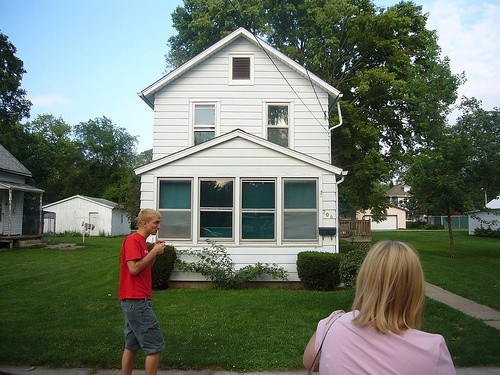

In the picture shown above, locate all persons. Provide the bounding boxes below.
[302,240,456,375]
[118,209,166,375]
